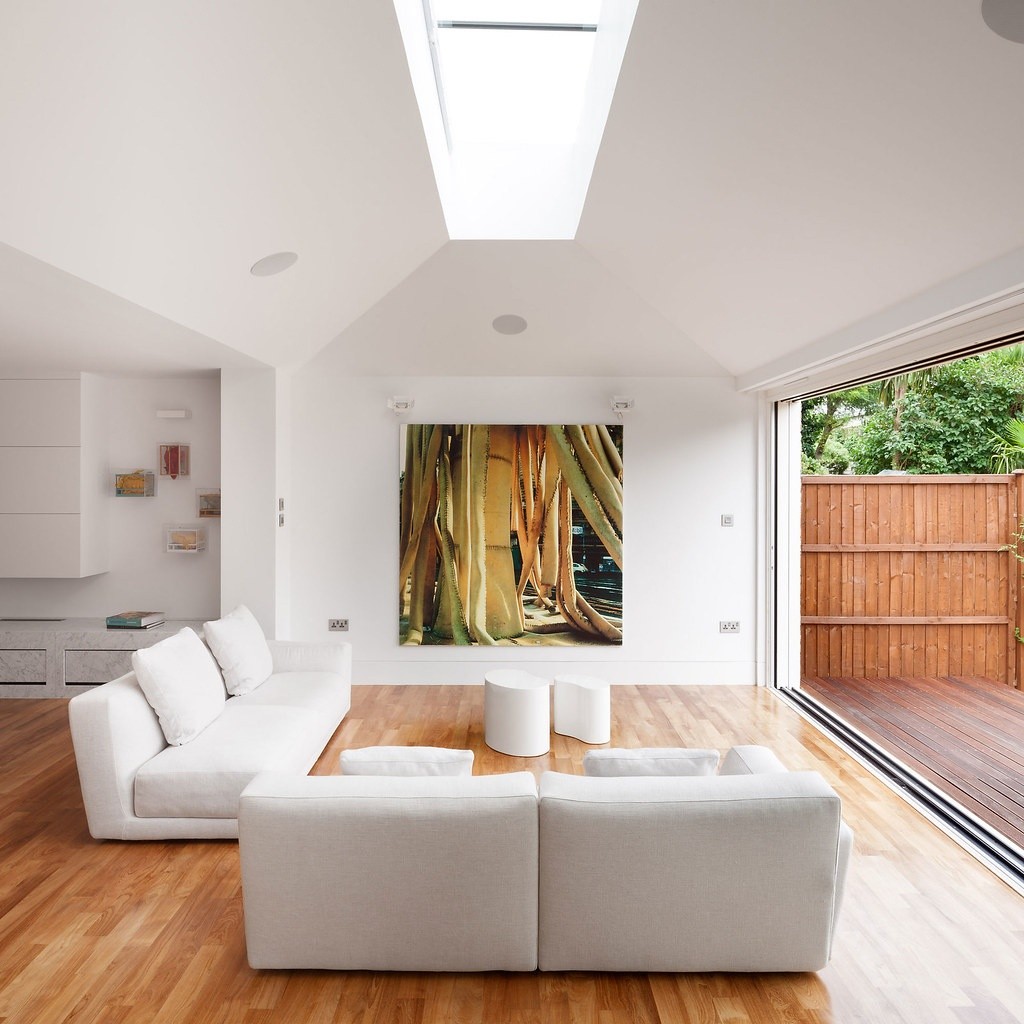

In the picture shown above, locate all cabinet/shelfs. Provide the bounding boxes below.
[0,380,108,578]
[1,615,221,699]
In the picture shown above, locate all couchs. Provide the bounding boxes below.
[67,636,353,840]
[237,745,855,972]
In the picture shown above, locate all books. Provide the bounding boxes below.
[106,612,165,627]
[107,621,165,629]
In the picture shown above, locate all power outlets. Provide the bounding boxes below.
[329,619,349,631]
[720,620,740,633]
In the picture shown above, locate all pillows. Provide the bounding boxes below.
[202,603,274,697]
[130,626,226,747]
[339,746,475,777]
[583,748,721,777]
